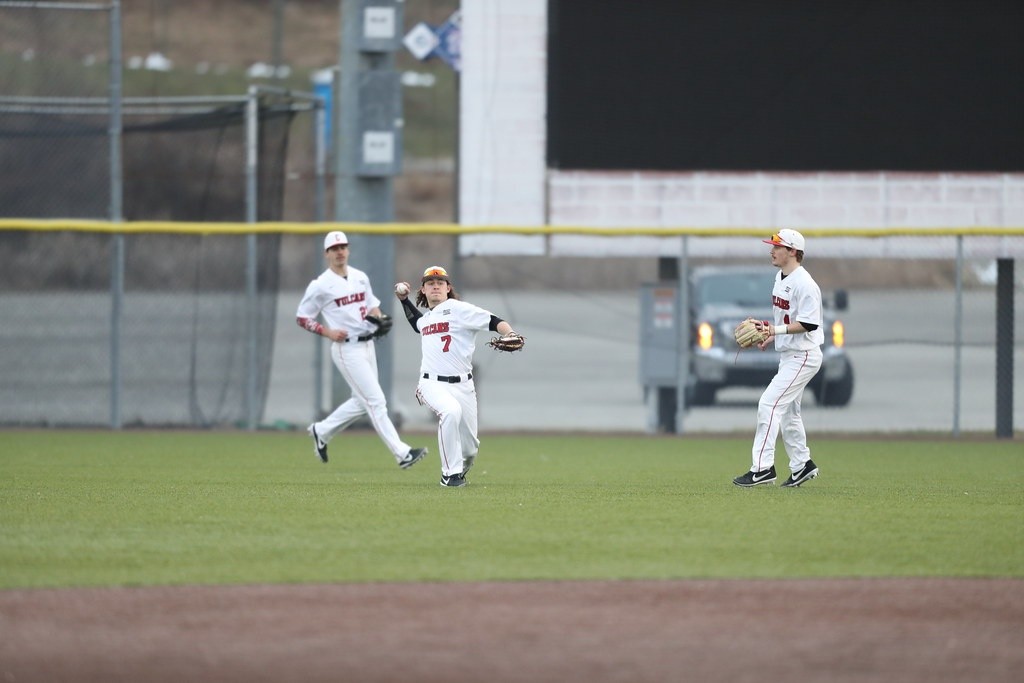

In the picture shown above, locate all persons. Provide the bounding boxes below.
[394,266,525,487]
[295,230,429,469]
[732,228,824,487]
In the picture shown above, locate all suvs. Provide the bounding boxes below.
[690,267,855,409]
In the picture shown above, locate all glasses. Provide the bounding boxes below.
[771,234,794,249]
[423,270,446,275]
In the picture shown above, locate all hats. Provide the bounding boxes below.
[762,229,804,252]
[422,266,448,282]
[324,231,348,251]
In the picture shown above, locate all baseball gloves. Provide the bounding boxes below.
[485,331,527,355]
[357,313,393,342]
[731,316,771,364]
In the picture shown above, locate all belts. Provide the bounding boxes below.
[422,373,472,383]
[345,334,372,342]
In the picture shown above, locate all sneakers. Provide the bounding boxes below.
[733,464,777,488]
[438,474,466,488]
[307,423,328,463]
[399,447,428,470]
[780,460,818,488]
[460,462,470,483]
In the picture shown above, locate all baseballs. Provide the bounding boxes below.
[396,282,408,295]
